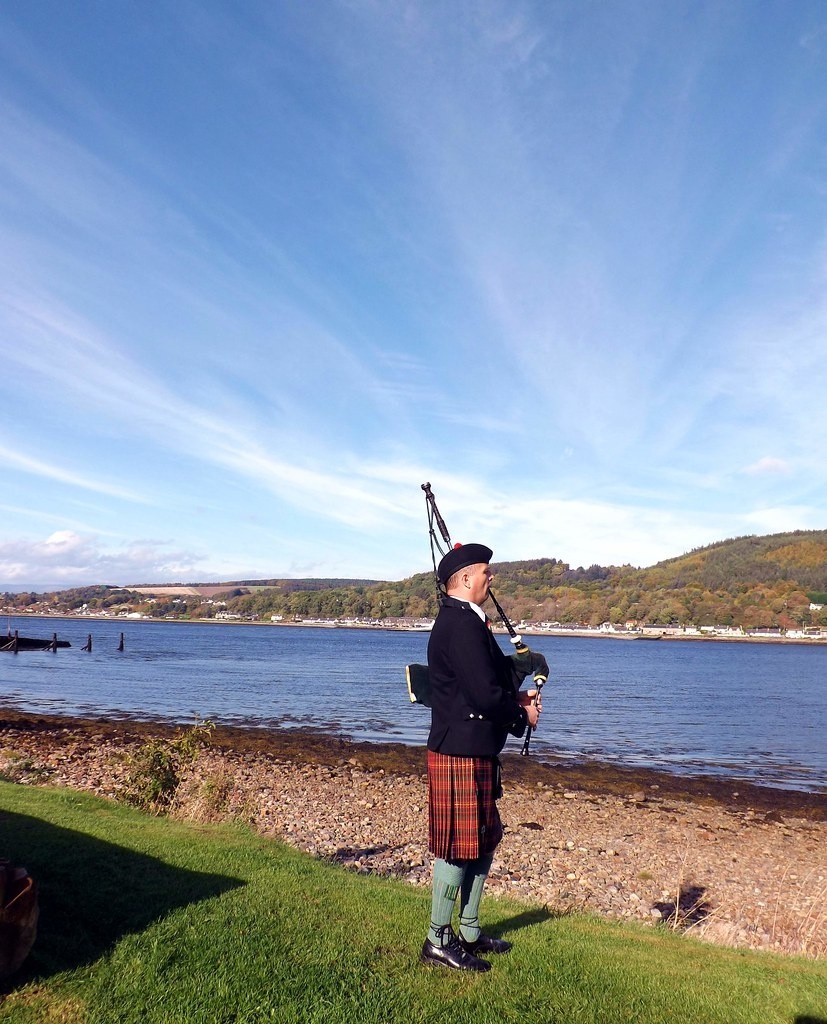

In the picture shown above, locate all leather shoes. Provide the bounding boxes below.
[457,932,513,955]
[421,932,492,973]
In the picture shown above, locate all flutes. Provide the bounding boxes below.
[422,482,551,757]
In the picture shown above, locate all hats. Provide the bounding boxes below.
[437,542,494,585]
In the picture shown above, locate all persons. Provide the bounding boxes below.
[406,543,542,973]
